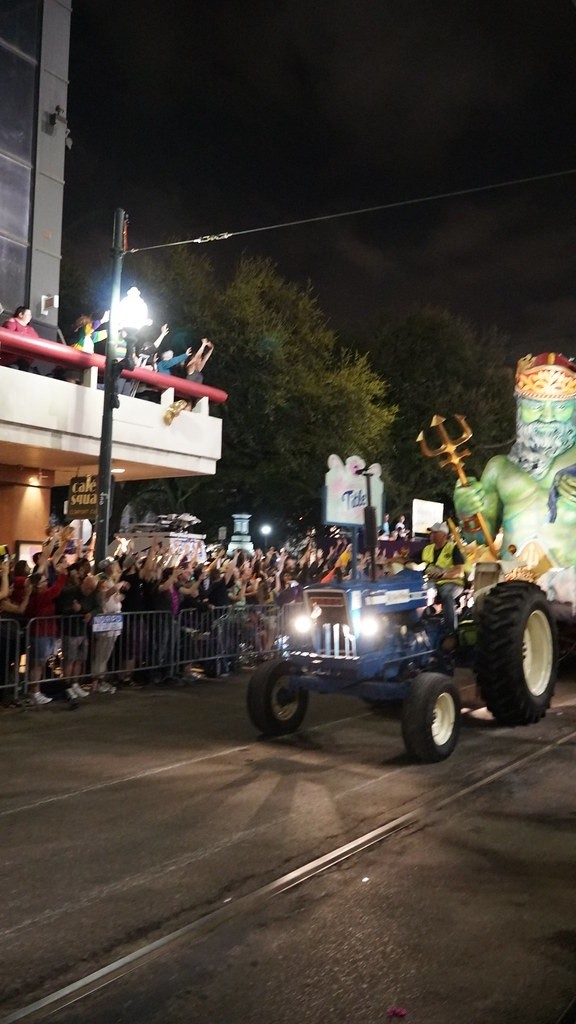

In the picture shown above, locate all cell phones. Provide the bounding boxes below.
[27,573,42,584]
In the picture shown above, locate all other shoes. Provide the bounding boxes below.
[92,680,117,695]
[442,637,456,650]
[71,682,90,697]
[64,688,79,700]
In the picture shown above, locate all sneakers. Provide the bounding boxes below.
[29,692,53,704]
[119,679,143,688]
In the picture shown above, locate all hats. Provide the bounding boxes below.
[427,522,449,534]
[98,560,115,570]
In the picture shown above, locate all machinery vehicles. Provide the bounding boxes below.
[247,468,576,763]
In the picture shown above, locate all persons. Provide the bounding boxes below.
[0,515,414,707]
[422,523,465,652]
[2,306,38,371]
[454,352,576,617]
[60,308,213,412]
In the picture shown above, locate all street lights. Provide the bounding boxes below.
[89,283,156,570]
